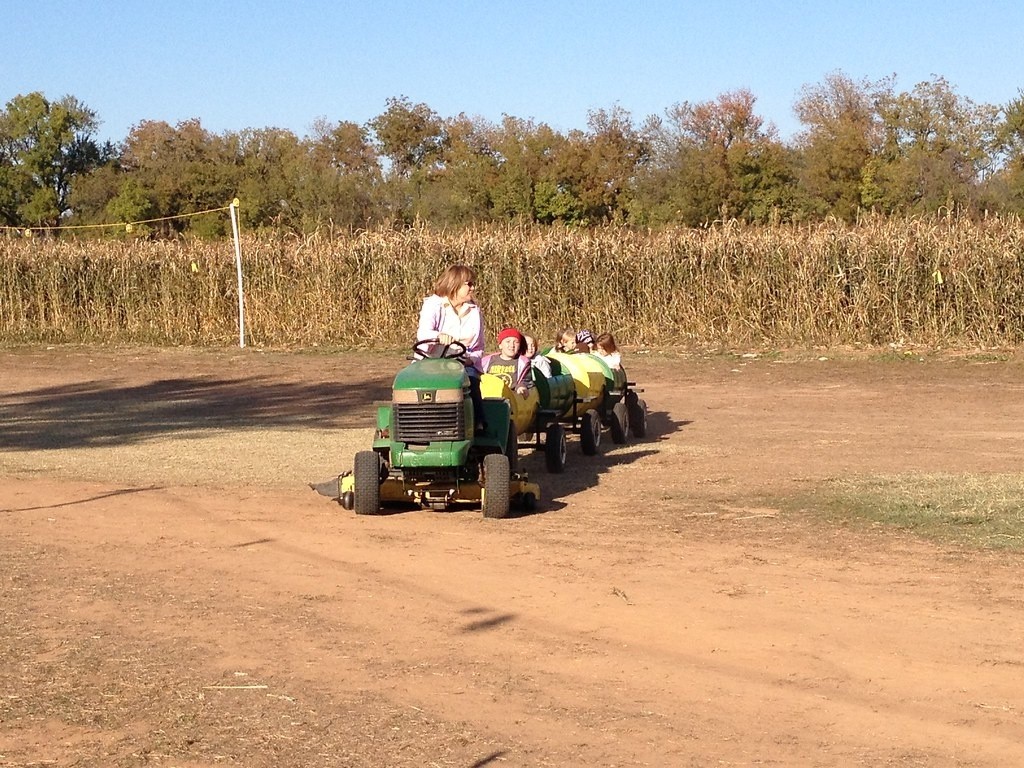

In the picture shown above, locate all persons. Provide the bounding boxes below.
[523,334,552,379]
[593,332,621,373]
[481,328,532,400]
[577,330,595,354]
[408,263,483,427]
[549,329,576,354]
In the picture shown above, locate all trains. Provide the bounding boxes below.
[307,337,650,519]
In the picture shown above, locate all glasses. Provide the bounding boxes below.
[462,282,475,287]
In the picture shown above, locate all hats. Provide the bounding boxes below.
[575,329,593,344]
[498,328,521,344]
